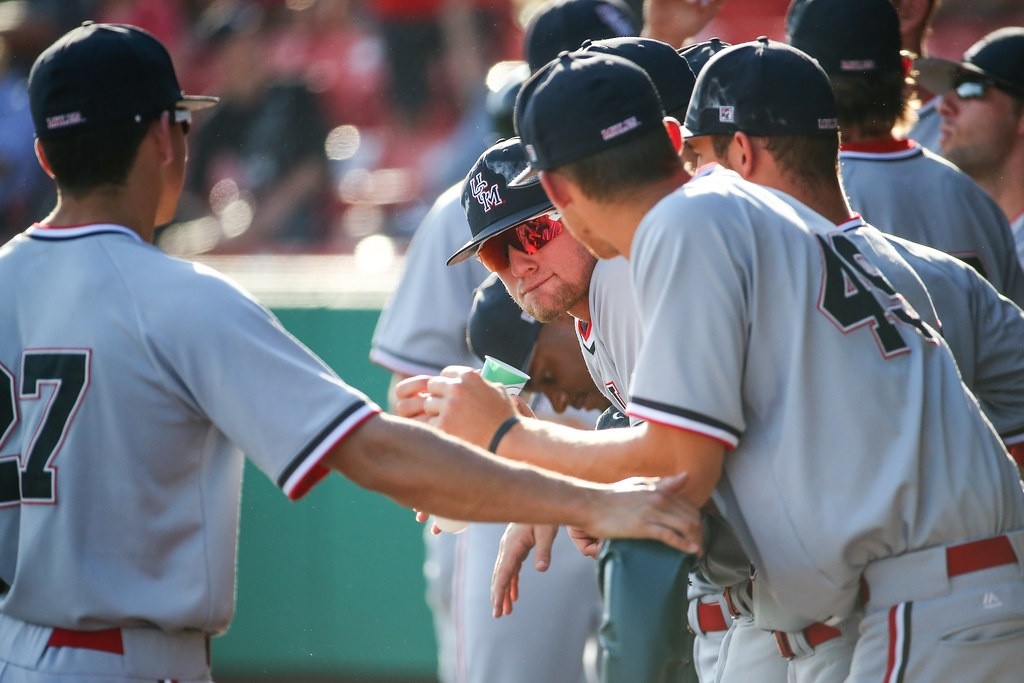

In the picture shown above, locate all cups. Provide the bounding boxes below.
[479,354,531,395]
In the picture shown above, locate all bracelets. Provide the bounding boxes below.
[488,417,519,454]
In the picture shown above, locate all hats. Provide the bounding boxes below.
[785,0,902,71]
[912,27,1024,94]
[679,35,840,139]
[676,36,732,77]
[576,36,697,113]
[446,136,555,268]
[26,20,220,138]
[508,51,663,188]
[501,0,645,110]
[467,271,544,374]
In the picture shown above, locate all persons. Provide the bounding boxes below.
[0,0,458,255]
[368,0,1024,683]
[0,17,706,683]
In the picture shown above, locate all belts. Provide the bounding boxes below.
[771,621,843,660]
[46,626,210,669]
[859,536,1019,602]
[697,580,752,634]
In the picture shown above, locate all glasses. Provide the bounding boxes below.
[167,106,192,134]
[949,78,1006,98]
[476,207,565,272]
